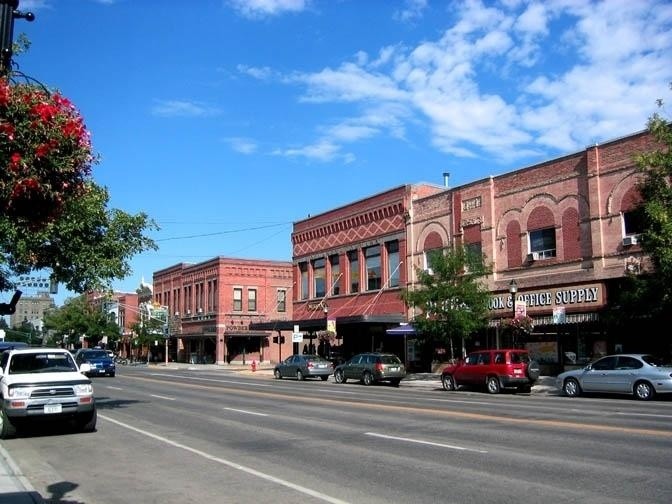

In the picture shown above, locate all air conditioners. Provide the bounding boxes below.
[526,252,540,262]
[622,235,637,246]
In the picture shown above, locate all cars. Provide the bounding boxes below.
[75,348,115,378]
[0,347,97,439]
[274,354,334,381]
[556,353,671,401]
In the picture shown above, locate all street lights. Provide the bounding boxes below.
[509,279,517,349]
[323,303,328,359]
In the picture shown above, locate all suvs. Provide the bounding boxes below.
[333,352,406,386]
[440,349,541,394]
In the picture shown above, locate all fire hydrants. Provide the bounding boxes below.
[249,360,255,372]
